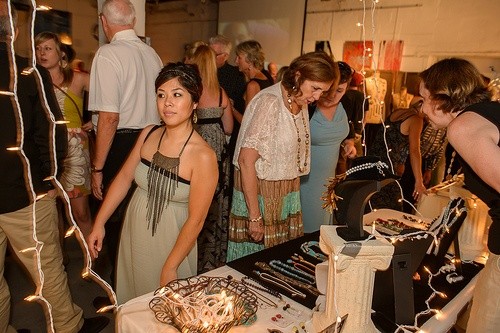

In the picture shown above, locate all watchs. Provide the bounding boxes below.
[90,165,105,174]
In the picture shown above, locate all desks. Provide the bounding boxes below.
[114,224,487,333]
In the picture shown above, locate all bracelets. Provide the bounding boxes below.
[249,214,263,223]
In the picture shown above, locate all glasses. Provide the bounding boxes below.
[185,43,195,50]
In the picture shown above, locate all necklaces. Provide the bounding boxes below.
[228,240,330,310]
[366,196,463,254]
[287,94,309,173]
[146,126,194,236]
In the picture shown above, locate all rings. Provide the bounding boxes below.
[416,192,419,195]
[413,190,415,192]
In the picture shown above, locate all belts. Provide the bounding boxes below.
[115,128,143,134]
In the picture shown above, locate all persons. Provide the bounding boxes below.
[299,62,356,235]
[87,1,164,313]
[0,0,110,333]
[342,73,369,156]
[209,32,290,148]
[88,62,219,307]
[372,95,428,212]
[419,58,500,333]
[35,32,90,281]
[182,41,235,275]
[226,51,341,262]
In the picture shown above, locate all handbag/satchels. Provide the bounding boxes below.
[86,130,96,167]
